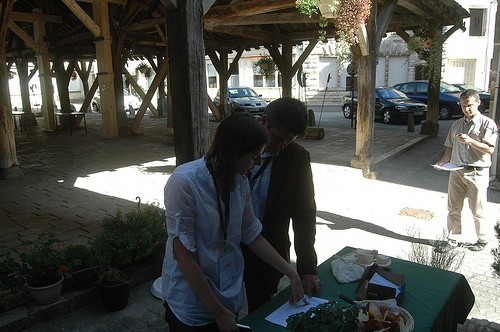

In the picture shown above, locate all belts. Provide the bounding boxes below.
[460,165,490,171]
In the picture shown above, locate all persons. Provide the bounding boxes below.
[162,113,310,332]
[435,89,498,250]
[241,98,320,312]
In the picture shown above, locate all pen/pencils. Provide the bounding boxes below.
[236,323,251,329]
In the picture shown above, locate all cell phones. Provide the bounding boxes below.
[456,133,463,137]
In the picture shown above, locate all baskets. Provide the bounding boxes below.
[351,299,415,332]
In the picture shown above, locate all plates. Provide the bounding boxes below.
[431,162,465,172]
[344,302,414,331]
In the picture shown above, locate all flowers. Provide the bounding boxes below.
[18,232,70,287]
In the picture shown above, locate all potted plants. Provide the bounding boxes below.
[64,245,97,283]
[90,205,169,267]
[97,267,132,313]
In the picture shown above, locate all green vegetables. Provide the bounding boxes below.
[286,300,360,332]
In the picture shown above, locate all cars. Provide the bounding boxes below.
[213,86,268,119]
[90,88,141,114]
[340,87,428,124]
[391,81,486,121]
[450,84,490,114]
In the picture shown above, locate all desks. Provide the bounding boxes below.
[55,111,87,136]
[236,246,475,332]
[12,110,31,133]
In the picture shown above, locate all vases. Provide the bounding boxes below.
[24,276,65,304]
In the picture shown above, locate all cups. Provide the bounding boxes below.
[366,283,396,300]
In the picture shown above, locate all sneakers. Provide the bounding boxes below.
[470,241,489,251]
[437,239,459,253]
